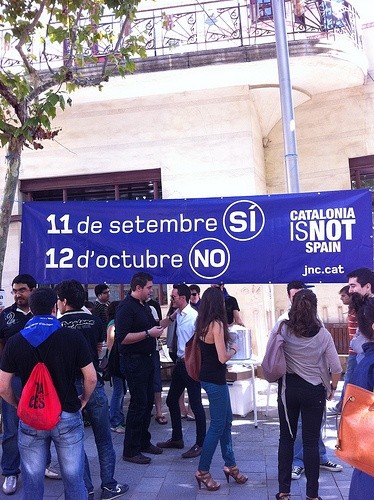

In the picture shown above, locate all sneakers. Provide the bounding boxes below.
[320,461,343,472]
[292,465,304,479]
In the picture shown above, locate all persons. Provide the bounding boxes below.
[338,266,374,500]
[0,286,99,500]
[271,279,345,480]
[186,286,249,490]
[264,289,344,500]
[45,278,130,500]
[93,270,247,464]
[0,273,63,494]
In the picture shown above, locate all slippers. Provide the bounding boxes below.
[155,415,168,425]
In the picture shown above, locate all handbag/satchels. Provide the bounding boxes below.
[100,356,111,382]
[260,320,285,383]
[333,383,374,477]
[183,328,201,382]
[108,337,126,379]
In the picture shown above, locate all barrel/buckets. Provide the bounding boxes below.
[226,327,252,360]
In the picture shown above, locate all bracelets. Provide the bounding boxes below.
[330,383,337,390]
[230,348,237,354]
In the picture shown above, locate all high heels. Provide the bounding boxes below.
[223,465,248,484]
[195,471,221,491]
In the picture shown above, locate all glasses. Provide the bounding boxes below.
[190,293,198,296]
[10,288,32,295]
[170,295,183,300]
[102,290,111,295]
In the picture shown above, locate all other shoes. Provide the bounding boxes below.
[44,467,62,480]
[110,426,125,434]
[122,453,151,464]
[157,439,185,449]
[275,493,292,500]
[182,444,202,457]
[141,444,162,454]
[305,496,323,500]
[100,484,129,500]
[2,476,17,496]
[87,488,94,495]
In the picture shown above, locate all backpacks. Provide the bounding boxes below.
[17,329,66,430]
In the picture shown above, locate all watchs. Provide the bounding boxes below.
[145,330,151,338]
